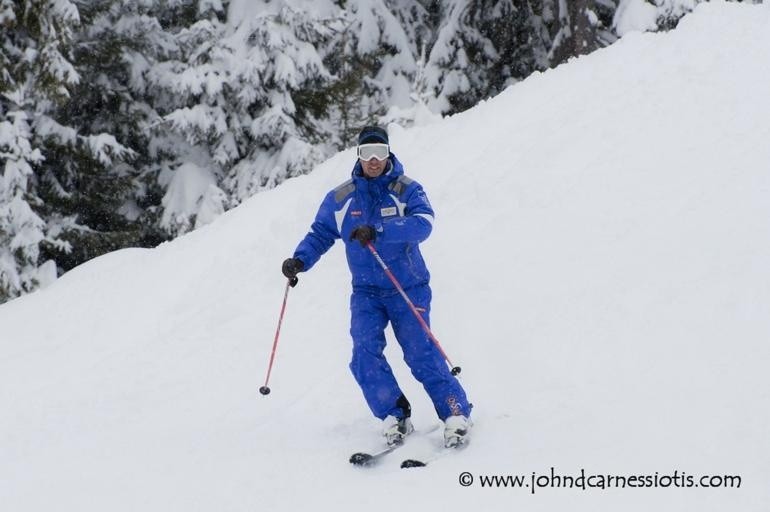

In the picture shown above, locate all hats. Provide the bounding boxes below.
[359,126,388,143]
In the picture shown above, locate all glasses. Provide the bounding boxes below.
[357,143,389,161]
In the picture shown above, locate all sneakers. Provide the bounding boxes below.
[444,415,473,447]
[384,416,414,445]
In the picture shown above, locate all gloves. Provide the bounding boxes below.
[282,258,304,279]
[348,225,376,247]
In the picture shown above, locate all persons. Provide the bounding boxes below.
[283,125,473,450]
